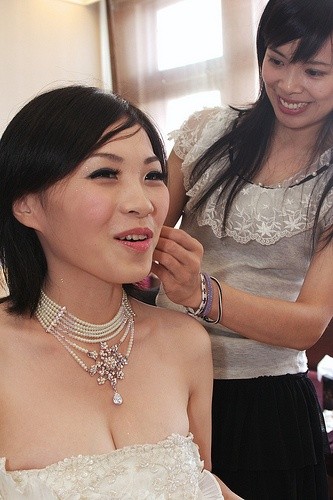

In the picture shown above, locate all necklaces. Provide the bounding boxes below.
[31,286,136,406]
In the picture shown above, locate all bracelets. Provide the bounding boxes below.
[188,272,224,324]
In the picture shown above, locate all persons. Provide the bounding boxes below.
[1,86,248,500]
[138,0,333,500]
